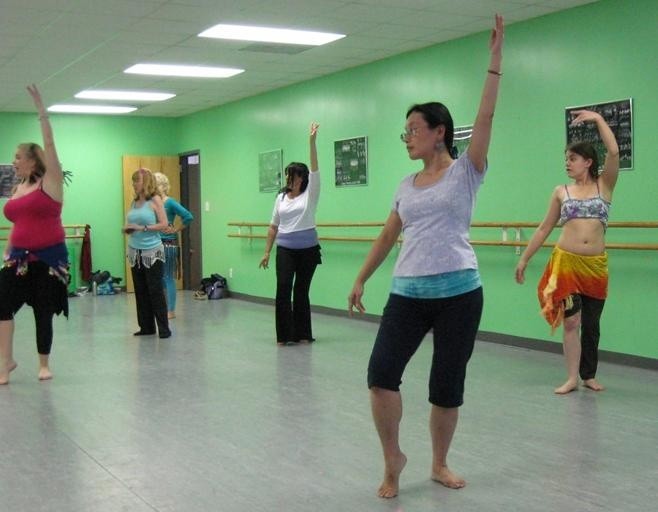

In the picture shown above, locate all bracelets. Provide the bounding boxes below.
[37,115,48,120]
[487,68,501,77]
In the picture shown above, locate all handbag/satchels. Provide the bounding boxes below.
[199,273,228,300]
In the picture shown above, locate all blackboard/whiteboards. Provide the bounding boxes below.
[565,98,634,172]
[453,125,473,159]
[258,149,282,192]
[334,136,369,187]
[0,165,21,196]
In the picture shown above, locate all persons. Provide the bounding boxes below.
[0,85,69,385]
[516,109,620,393]
[259,122,319,345]
[347,14,503,500]
[153,173,194,320]
[121,169,170,339]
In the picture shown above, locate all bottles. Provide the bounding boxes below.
[92,281,97,296]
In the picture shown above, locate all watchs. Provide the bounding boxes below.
[143,225,148,232]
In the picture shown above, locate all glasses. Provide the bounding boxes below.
[398,126,419,141]
